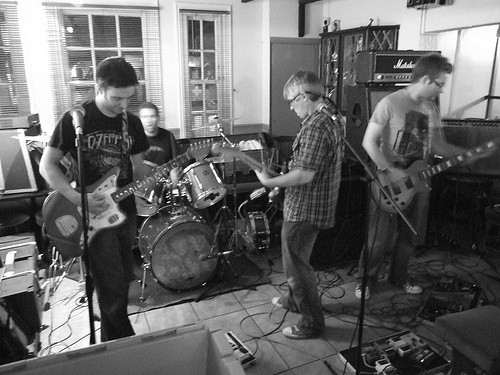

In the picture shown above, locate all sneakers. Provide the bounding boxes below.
[354,283,370,301]
[387,274,424,294]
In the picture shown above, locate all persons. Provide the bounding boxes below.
[355,54,469,298]
[39,57,182,340]
[139,102,178,166]
[254,70,341,339]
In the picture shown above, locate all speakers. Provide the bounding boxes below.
[345,84,407,174]
[441,119,500,176]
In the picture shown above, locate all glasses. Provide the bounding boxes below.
[287,93,300,106]
[430,76,445,89]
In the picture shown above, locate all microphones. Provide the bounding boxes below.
[199,250,233,262]
[317,103,338,121]
[69,105,86,136]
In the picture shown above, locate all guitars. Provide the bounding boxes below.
[370,135,500,213]
[42,137,213,256]
[210,140,288,204]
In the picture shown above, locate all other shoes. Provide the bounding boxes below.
[282,325,306,340]
[271,296,283,308]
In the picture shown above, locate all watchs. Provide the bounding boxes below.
[380,167,395,175]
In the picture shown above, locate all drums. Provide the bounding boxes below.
[247,212,271,252]
[133,159,171,205]
[178,159,228,209]
[140,201,220,291]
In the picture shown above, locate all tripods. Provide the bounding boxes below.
[210,118,274,264]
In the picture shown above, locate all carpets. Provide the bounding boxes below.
[125,252,272,316]
